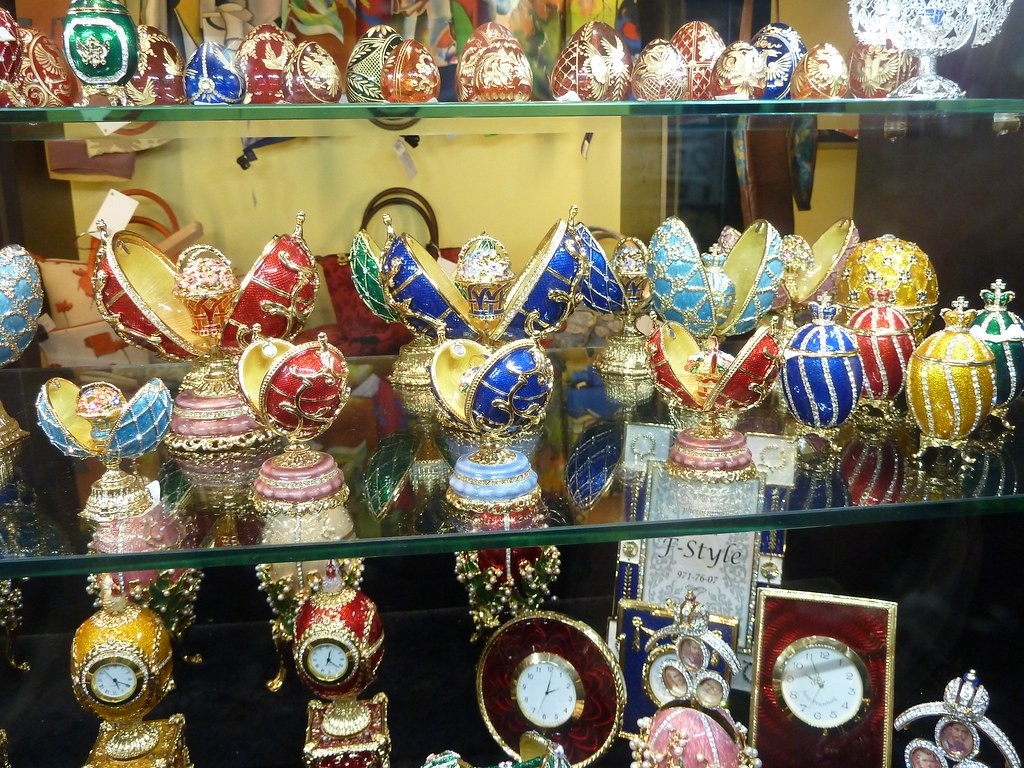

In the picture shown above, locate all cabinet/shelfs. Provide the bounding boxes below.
[0,0,1024,768]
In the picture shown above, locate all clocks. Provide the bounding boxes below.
[307,643,349,681]
[773,636,872,736]
[93,663,139,700]
[511,651,587,738]
